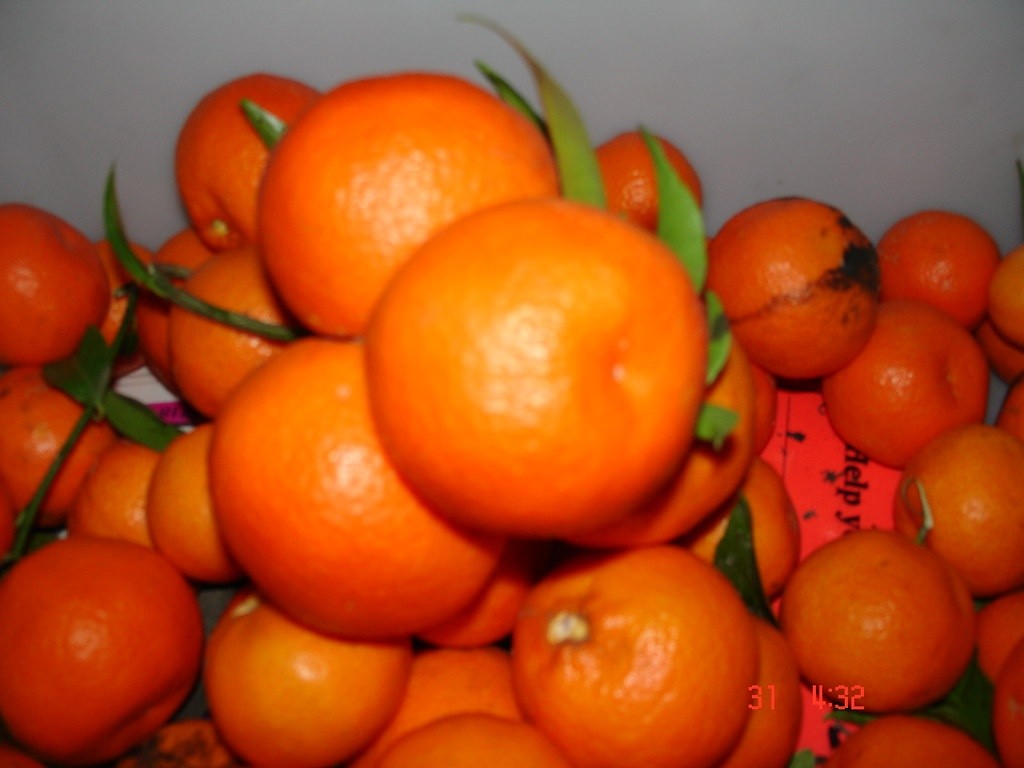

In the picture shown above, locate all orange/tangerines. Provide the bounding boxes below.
[0,17,1024,768]
